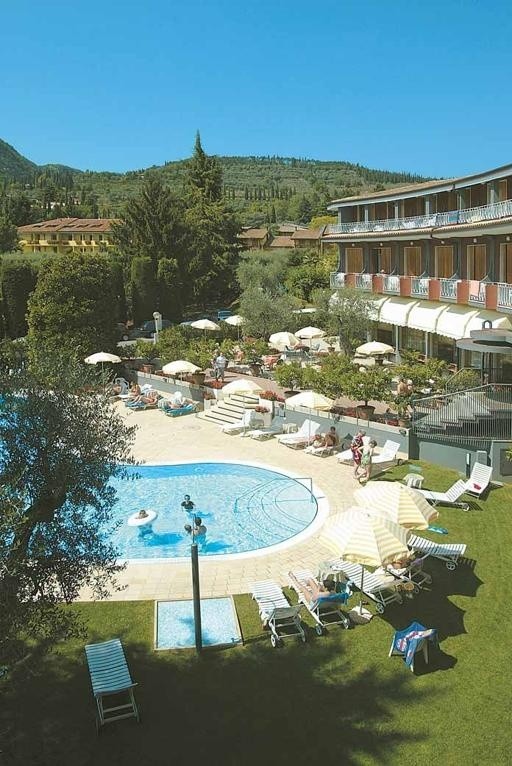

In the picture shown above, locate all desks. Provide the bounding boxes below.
[404,472,425,487]
[316,561,343,591]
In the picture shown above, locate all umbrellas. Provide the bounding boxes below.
[285,392,336,446]
[191,319,221,342]
[269,325,327,365]
[162,360,203,392]
[225,315,251,341]
[222,378,263,433]
[85,351,121,395]
[354,340,396,360]
[319,480,439,615]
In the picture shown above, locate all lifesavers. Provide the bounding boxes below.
[127,509,157,528]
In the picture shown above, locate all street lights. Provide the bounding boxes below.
[191,513,202,652]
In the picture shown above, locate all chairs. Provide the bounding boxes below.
[414,478,471,512]
[408,533,467,571]
[85,638,142,733]
[388,622,437,672]
[117,384,200,416]
[219,408,400,485]
[387,553,432,593]
[253,579,307,646]
[465,462,493,499]
[287,568,350,634]
[334,557,406,613]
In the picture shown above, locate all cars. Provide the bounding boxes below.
[115,320,172,340]
[217,310,231,321]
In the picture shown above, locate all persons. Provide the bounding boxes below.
[184,517,207,537]
[128,380,159,405]
[351,430,377,479]
[111,379,122,395]
[181,495,195,510]
[288,571,351,608]
[169,397,194,409]
[313,426,338,449]
[138,509,148,519]
[397,377,417,421]
[213,349,226,383]
